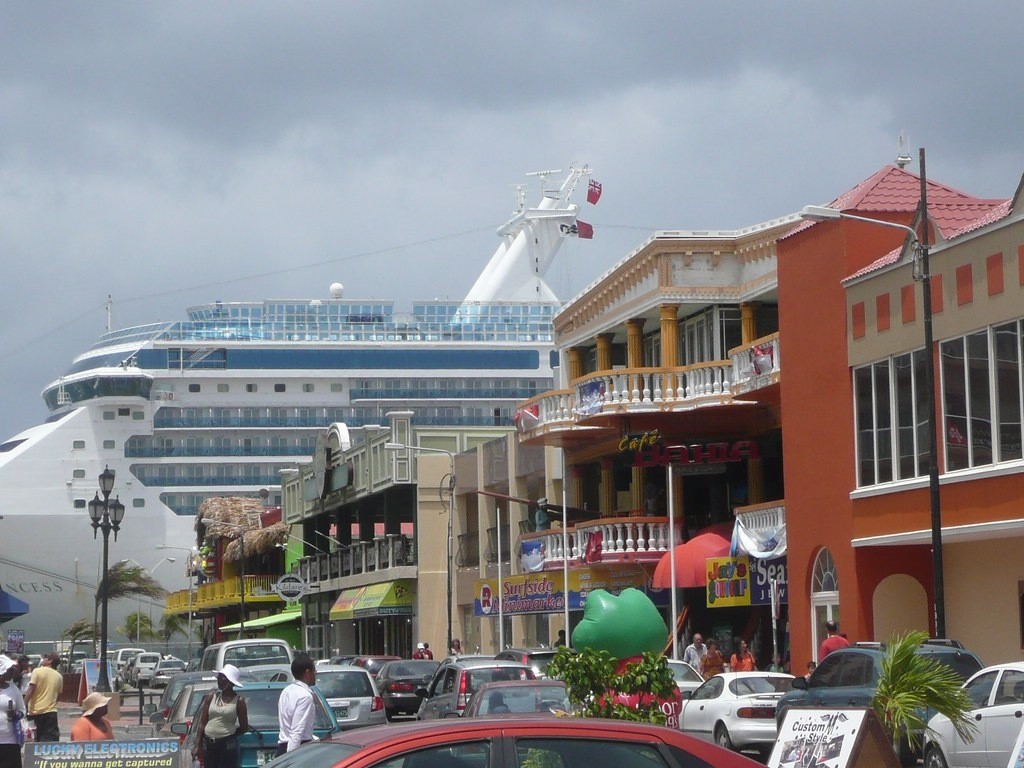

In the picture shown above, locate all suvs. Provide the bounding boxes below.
[776,639,986,768]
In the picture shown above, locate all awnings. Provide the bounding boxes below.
[652,542,719,589]
[0,590,30,624]
[328,580,413,621]
[219,613,302,632]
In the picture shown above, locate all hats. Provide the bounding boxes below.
[212,664,243,688]
[81,692,112,716]
[44,652,61,664]
[418,643,425,649]
[0,655,17,675]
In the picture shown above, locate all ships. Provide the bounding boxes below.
[0,162,602,660]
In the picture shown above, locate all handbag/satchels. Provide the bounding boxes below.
[13,719,32,746]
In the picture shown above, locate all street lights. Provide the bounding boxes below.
[121,558,176,652]
[384,443,455,657]
[200,518,245,640]
[88,462,125,693]
[155,544,193,662]
[798,148,946,645]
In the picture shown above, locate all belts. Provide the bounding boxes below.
[203,733,236,744]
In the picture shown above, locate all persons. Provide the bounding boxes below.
[764,653,784,672]
[554,630,572,648]
[697,638,724,682]
[0,649,31,768]
[535,498,553,532]
[803,662,816,681]
[729,639,758,671]
[192,663,248,768]
[71,692,114,740]
[276,656,317,759]
[451,638,463,654]
[25,651,63,742]
[818,621,850,661]
[412,643,433,660]
[684,634,708,670]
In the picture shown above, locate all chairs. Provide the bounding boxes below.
[540,701,565,711]
[493,705,511,712]
[1013,681,1024,698]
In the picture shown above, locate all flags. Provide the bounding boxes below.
[586,531,603,562]
[577,220,594,239]
[587,179,602,205]
[521,541,544,574]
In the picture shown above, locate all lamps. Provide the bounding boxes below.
[283,533,326,554]
[275,541,304,559]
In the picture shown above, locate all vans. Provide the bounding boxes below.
[55,652,89,671]
[195,639,295,671]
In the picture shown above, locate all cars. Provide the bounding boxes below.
[922,660,1024,768]
[98,648,197,689]
[144,664,340,768]
[316,647,706,730]
[261,714,769,768]
[26,654,44,667]
[678,671,796,755]
